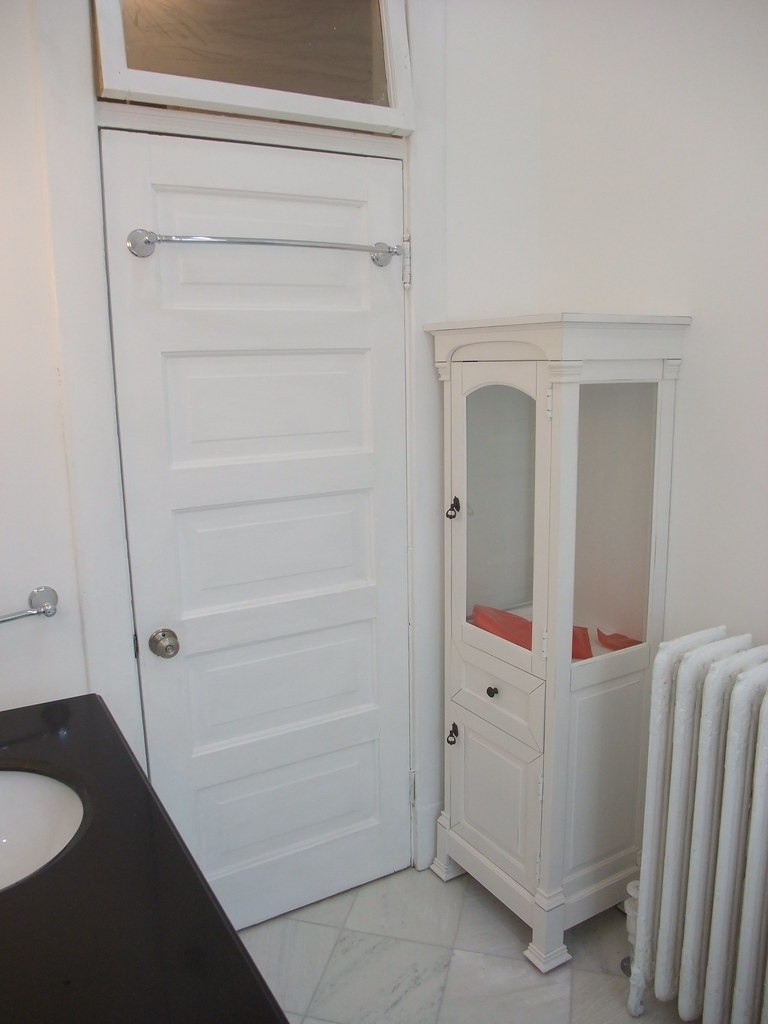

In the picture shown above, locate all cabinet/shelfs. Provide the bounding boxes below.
[425,311,695,974]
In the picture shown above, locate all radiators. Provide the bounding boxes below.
[622,627,767,1024]
